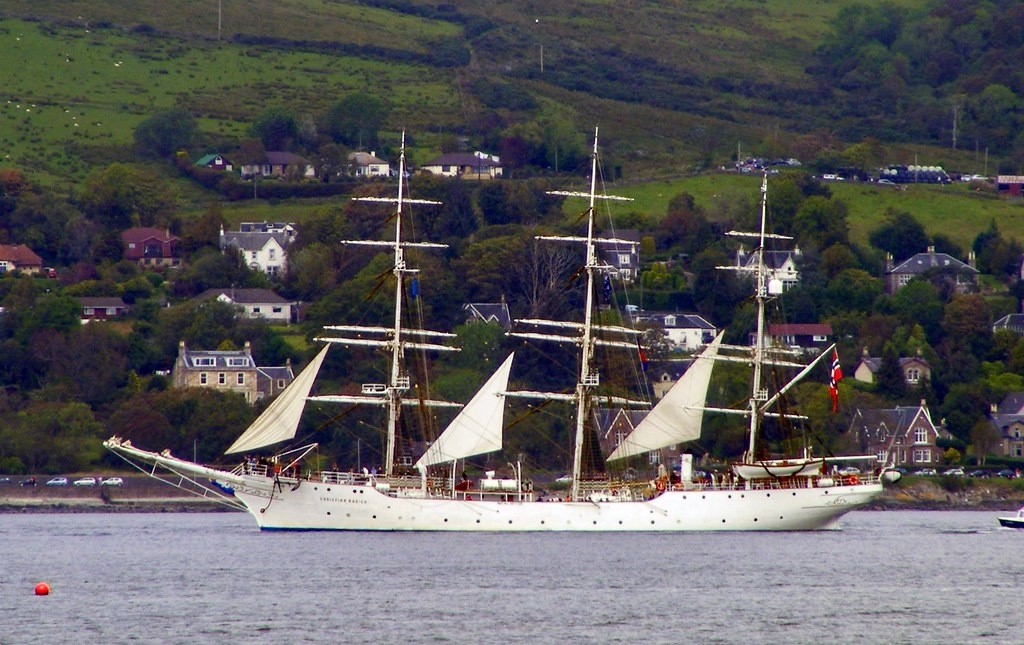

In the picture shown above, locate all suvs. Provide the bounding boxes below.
[943,466,964,478]
[73,478,95,487]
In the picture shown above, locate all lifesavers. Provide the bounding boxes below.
[657,482,666,490]
[849,476,858,485]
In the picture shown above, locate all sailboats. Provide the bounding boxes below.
[98,121,910,534]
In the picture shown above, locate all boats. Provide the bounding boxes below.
[998,507,1024,528]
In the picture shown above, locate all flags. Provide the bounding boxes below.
[829,346,843,415]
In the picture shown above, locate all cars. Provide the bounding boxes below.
[913,468,936,475]
[46,477,67,487]
[894,468,907,475]
[19,477,38,487]
[617,473,637,482]
[838,465,861,476]
[995,470,1016,478]
[102,477,123,487]
[0,478,11,486]
[968,470,991,479]
[863,468,874,476]
[554,475,572,484]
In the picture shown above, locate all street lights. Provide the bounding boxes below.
[194,439,197,487]
[357,439,361,472]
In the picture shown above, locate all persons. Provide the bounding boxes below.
[331,460,339,479]
[718,473,722,485]
[259,456,270,474]
[295,463,301,479]
[461,470,466,481]
[243,454,251,475]
[344,465,447,485]
[727,467,734,482]
[465,494,610,502]
[670,470,676,484]
[288,458,295,477]
[252,455,260,471]
[706,470,712,487]
